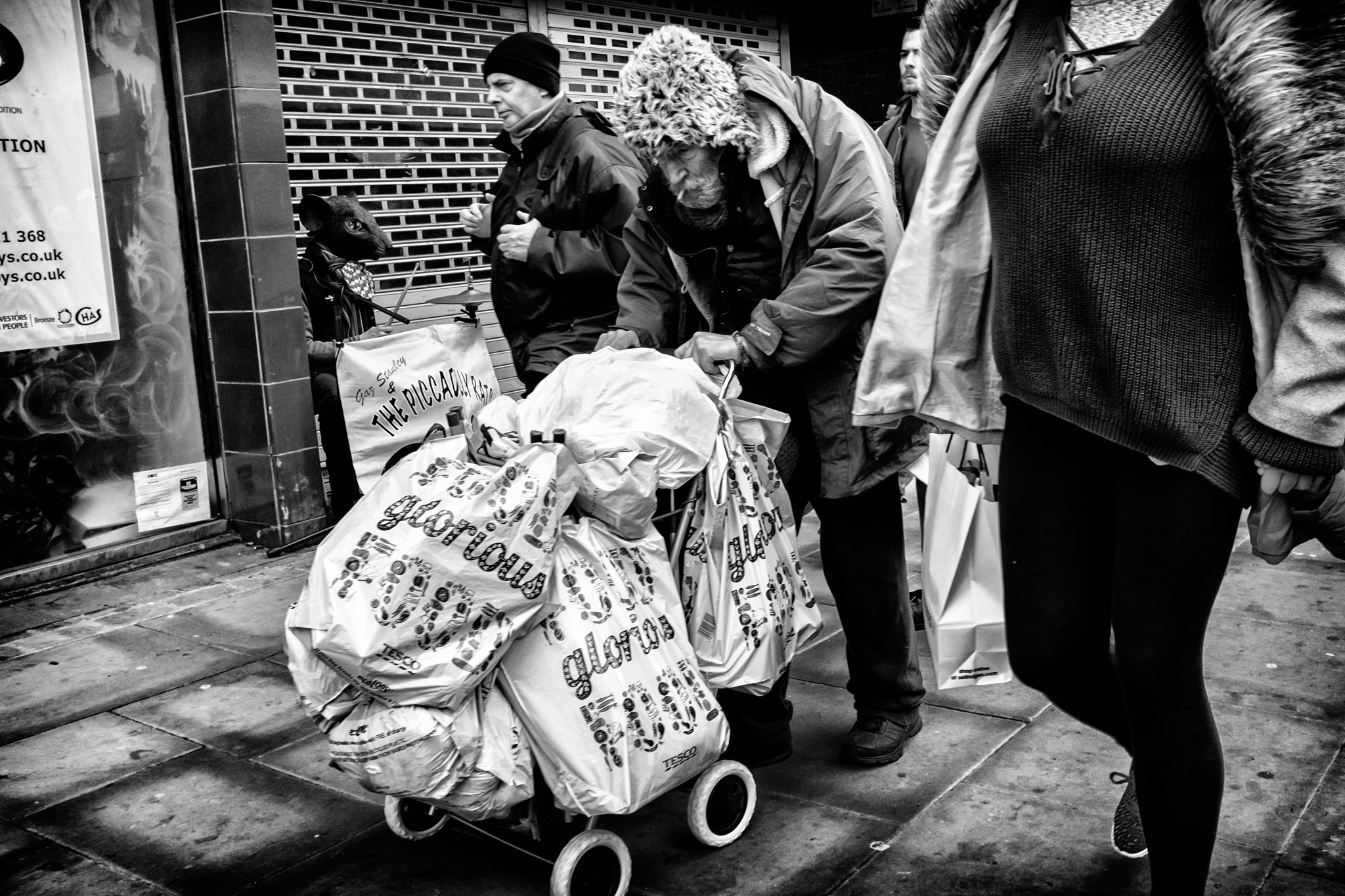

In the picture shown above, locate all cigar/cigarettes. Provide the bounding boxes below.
[675,190,686,203]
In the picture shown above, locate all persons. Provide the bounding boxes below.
[855,0,1345,896]
[297,191,395,513]
[462,32,647,396]
[874,23,928,231]
[595,26,925,791]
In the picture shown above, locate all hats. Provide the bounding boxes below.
[482,33,562,97]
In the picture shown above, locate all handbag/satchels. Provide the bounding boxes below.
[681,388,820,697]
[284,341,743,819]
[920,427,1014,690]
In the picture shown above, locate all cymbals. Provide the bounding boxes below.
[426,290,492,304]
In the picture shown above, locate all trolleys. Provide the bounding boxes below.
[383,355,758,895]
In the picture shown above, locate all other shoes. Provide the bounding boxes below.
[1109,760,1150,859]
[842,708,924,766]
[680,716,792,794]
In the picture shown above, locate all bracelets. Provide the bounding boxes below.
[732,331,750,367]
